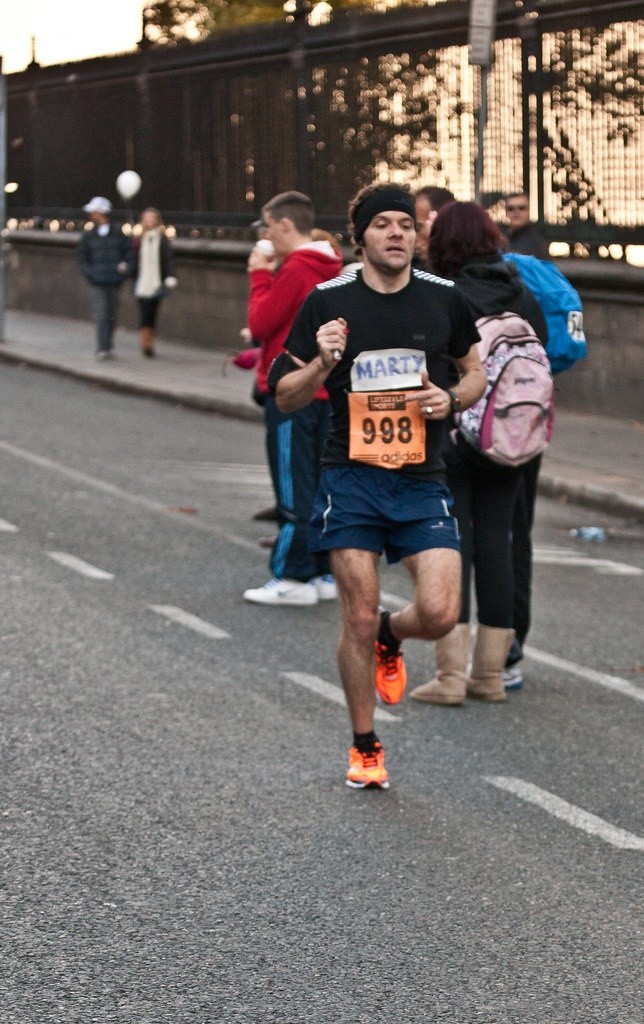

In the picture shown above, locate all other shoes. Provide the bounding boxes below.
[261,538,277,549]
[502,666,523,688]
[255,507,278,520]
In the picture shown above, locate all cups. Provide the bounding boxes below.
[246,240,275,274]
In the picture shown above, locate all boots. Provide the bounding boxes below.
[408,623,469,705]
[466,624,513,702]
[139,327,153,356]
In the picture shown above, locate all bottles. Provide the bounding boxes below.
[570,527,614,542]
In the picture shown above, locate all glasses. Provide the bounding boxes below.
[507,204,527,211]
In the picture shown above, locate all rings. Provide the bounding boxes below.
[426,406,433,415]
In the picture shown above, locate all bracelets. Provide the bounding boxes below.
[447,390,460,414]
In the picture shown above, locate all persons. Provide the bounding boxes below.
[117,207,179,356]
[233,190,342,606]
[75,196,135,360]
[409,185,543,704]
[266,182,487,790]
[503,192,554,262]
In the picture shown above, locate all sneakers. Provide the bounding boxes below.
[308,574,338,600]
[243,576,318,606]
[346,739,390,789]
[374,606,407,704]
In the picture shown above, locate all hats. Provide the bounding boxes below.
[82,196,113,216]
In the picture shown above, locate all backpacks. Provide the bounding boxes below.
[501,252,587,375]
[448,286,562,468]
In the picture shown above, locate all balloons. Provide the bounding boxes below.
[116,170,141,198]
